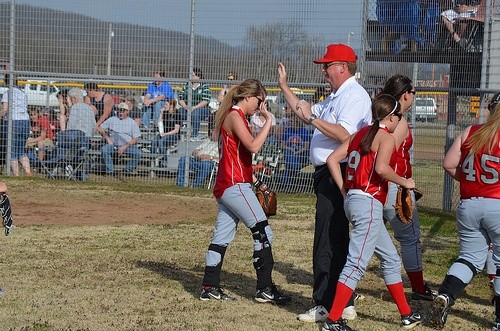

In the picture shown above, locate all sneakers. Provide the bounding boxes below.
[296,304,328,322]
[322,318,353,331]
[198,288,236,301]
[255,283,291,304]
[341,306,357,320]
[401,309,423,328]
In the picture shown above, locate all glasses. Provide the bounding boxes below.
[228,79,232,80]
[324,62,345,70]
[396,112,402,122]
[410,91,416,95]
[256,97,262,107]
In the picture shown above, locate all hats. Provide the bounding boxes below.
[116,103,129,111]
[313,44,357,64]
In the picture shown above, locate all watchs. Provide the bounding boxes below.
[308,114,317,123]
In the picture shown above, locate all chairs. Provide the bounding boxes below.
[39,129,89,182]
[434,0,484,54]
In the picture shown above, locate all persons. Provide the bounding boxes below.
[440,0,480,53]
[378,74,438,300]
[321,94,426,331]
[0,68,333,193]
[199,79,291,303]
[278,44,386,323]
[426,92,500,331]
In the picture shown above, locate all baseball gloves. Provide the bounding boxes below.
[395,183,416,222]
[254,180,279,217]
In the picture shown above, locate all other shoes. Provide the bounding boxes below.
[412,288,438,300]
[353,293,364,300]
[492,322,500,331]
[433,293,450,328]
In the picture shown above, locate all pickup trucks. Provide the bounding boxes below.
[0,79,62,110]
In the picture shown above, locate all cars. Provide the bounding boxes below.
[407,96,441,121]
